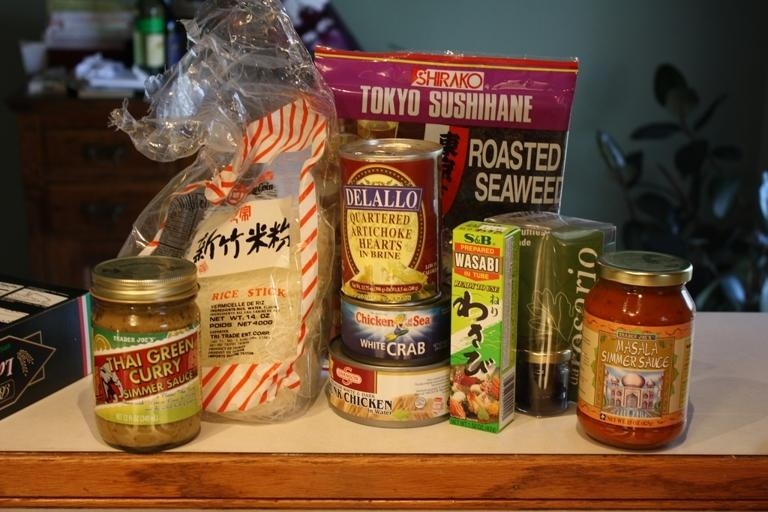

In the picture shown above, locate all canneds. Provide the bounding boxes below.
[86,254,204,452]
[516,347,572,419]
[325,284,450,429]
[575,248,697,449]
[337,138,443,302]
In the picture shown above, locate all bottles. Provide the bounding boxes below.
[132,0,167,76]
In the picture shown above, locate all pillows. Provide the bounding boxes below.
[5,67,199,286]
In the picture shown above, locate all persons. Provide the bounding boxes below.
[0,1,52,279]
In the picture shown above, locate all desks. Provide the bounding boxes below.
[1,309,768,507]
